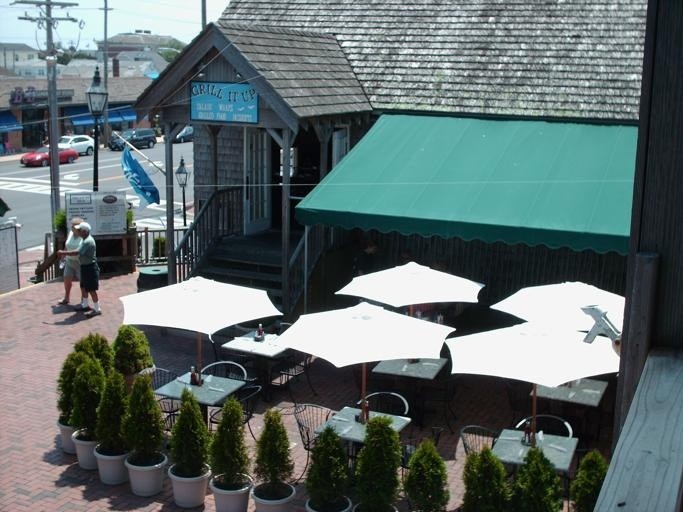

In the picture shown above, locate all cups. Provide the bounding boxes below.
[437,315,444,325]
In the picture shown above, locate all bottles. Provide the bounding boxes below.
[59,258,66,269]
[364,401,369,421]
[190,366,196,384]
[258,324,264,336]
[523,420,532,443]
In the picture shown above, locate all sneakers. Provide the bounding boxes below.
[58,298,69,304]
[74,304,101,316]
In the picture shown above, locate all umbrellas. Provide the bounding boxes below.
[268,302,457,426]
[333,261,485,362]
[119,275,283,386]
[443,317,620,448]
[488,280,626,388]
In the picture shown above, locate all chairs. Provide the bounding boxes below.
[459,425,498,461]
[207,325,319,404]
[349,359,458,435]
[139,365,182,430]
[400,425,447,477]
[209,384,262,446]
[294,404,335,485]
[199,359,249,424]
[516,413,574,439]
[356,391,411,418]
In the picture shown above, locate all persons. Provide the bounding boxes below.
[57,216,83,304]
[57,222,101,317]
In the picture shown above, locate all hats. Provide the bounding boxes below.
[72,217,92,232]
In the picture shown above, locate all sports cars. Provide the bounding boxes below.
[20,145,79,167]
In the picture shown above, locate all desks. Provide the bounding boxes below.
[490,426,580,473]
[153,371,246,407]
[315,406,412,446]
[528,379,608,408]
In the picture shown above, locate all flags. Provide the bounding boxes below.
[121,144,159,211]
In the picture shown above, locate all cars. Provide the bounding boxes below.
[175,125,194,143]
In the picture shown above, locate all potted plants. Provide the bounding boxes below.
[57,351,89,456]
[351,415,404,512]
[124,371,168,497]
[250,407,298,512]
[303,422,352,512]
[70,358,113,471]
[166,383,212,509]
[206,395,255,512]
[94,373,135,486]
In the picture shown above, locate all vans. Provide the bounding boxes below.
[107,127,157,152]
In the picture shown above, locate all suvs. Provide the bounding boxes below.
[44,134,94,156]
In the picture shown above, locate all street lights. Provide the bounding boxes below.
[175,154,191,261]
[85,64,108,191]
